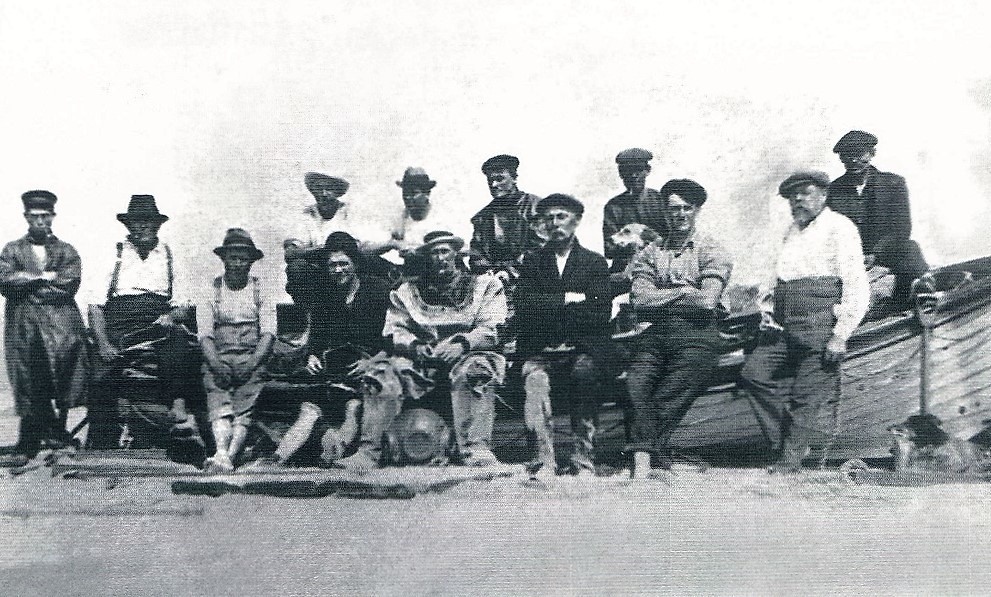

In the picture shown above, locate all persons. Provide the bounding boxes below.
[619,177,735,484]
[512,191,615,489]
[825,130,930,328]
[282,169,378,350]
[469,153,553,352]
[333,225,511,473]
[353,164,473,287]
[736,168,871,474]
[0,188,92,468]
[86,192,208,451]
[254,231,393,472]
[194,227,278,475]
[601,148,672,384]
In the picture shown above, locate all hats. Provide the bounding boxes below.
[832,130,878,153]
[317,231,368,264]
[537,193,583,215]
[22,190,58,212]
[214,228,265,260]
[615,148,651,165]
[118,193,169,223]
[396,166,438,189]
[305,172,350,196]
[482,154,520,174]
[416,230,464,251]
[779,170,830,198]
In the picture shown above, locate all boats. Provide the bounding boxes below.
[84,253,991,483]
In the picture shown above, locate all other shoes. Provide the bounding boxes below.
[333,451,377,472]
[459,443,499,465]
